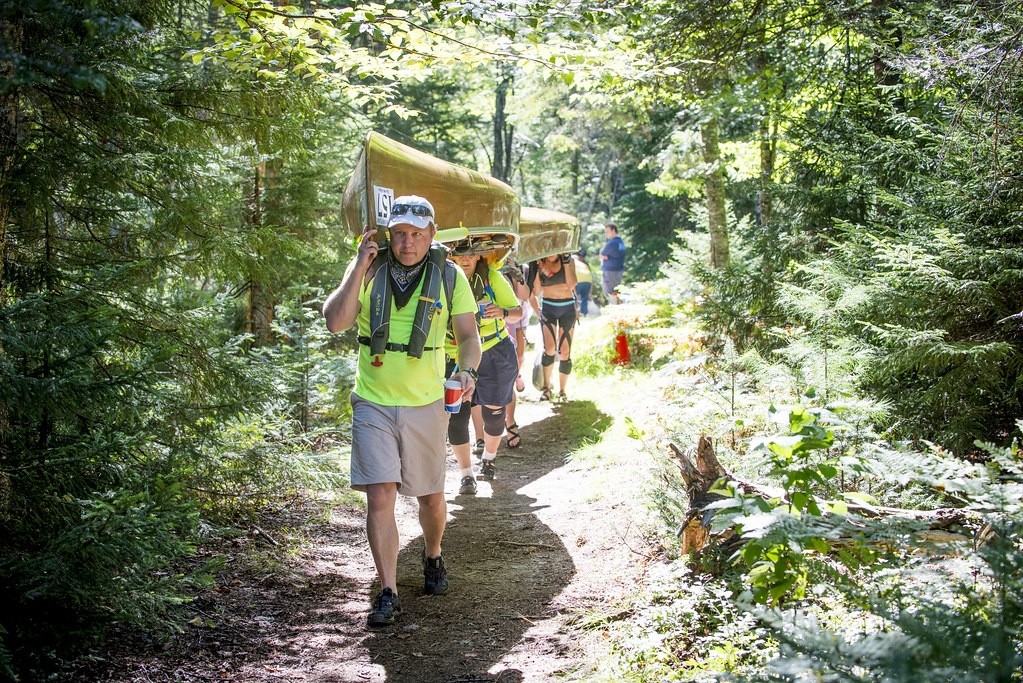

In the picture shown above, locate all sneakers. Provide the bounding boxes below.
[420,547,449,594]
[515,375,524,392]
[458,475,477,496]
[366,588,403,625]
[477,455,496,480]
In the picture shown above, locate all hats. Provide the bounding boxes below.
[387,195,435,229]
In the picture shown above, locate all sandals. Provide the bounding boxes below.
[540,387,550,400]
[504,420,520,448]
[557,393,566,404]
[472,438,486,455]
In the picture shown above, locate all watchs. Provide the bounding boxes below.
[459,367,478,384]
[501,307,509,320]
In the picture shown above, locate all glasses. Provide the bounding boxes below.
[389,204,433,218]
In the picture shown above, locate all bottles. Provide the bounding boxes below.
[537,260,553,278]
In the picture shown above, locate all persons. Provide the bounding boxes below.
[532,253,578,405]
[571,249,592,318]
[442,240,530,494]
[322,194,482,627]
[598,223,625,305]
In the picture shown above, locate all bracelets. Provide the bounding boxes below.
[515,278,523,282]
[561,255,570,263]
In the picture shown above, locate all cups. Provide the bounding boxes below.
[443,381,462,413]
[477,300,492,319]
